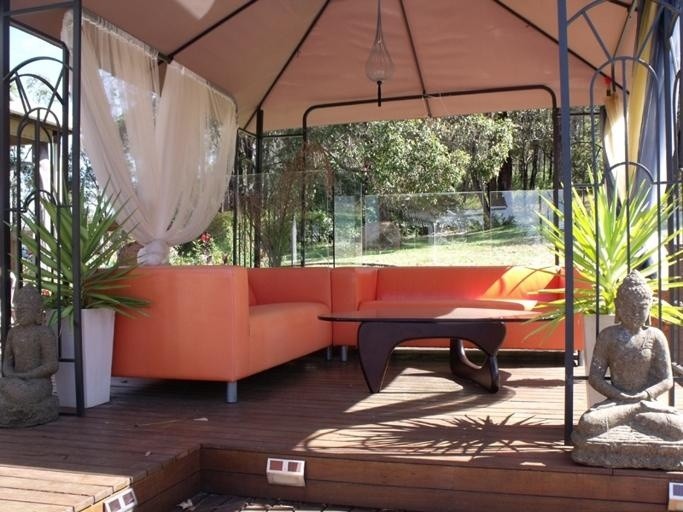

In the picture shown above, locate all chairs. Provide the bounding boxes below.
[90,265,335,403]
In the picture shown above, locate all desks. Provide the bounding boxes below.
[317,308,560,396]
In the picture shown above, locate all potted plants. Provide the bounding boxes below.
[519,161,683,408]
[1,171,154,409]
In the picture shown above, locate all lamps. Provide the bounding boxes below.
[365,0,393,107]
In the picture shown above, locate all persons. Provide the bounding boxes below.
[0,277,59,403]
[579,269,683,438]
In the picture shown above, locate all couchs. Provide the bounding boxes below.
[327,264,592,368]
